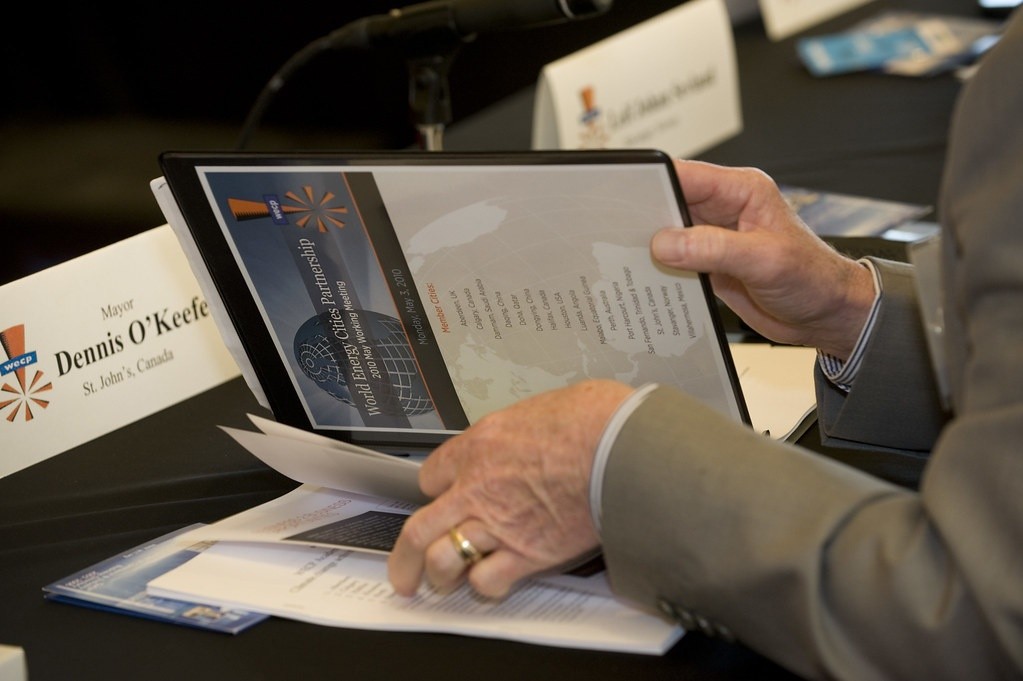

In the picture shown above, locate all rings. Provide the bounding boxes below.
[450,528,479,566]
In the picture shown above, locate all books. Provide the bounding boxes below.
[152,148,835,457]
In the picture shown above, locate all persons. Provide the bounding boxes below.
[384,6,1022,681]
